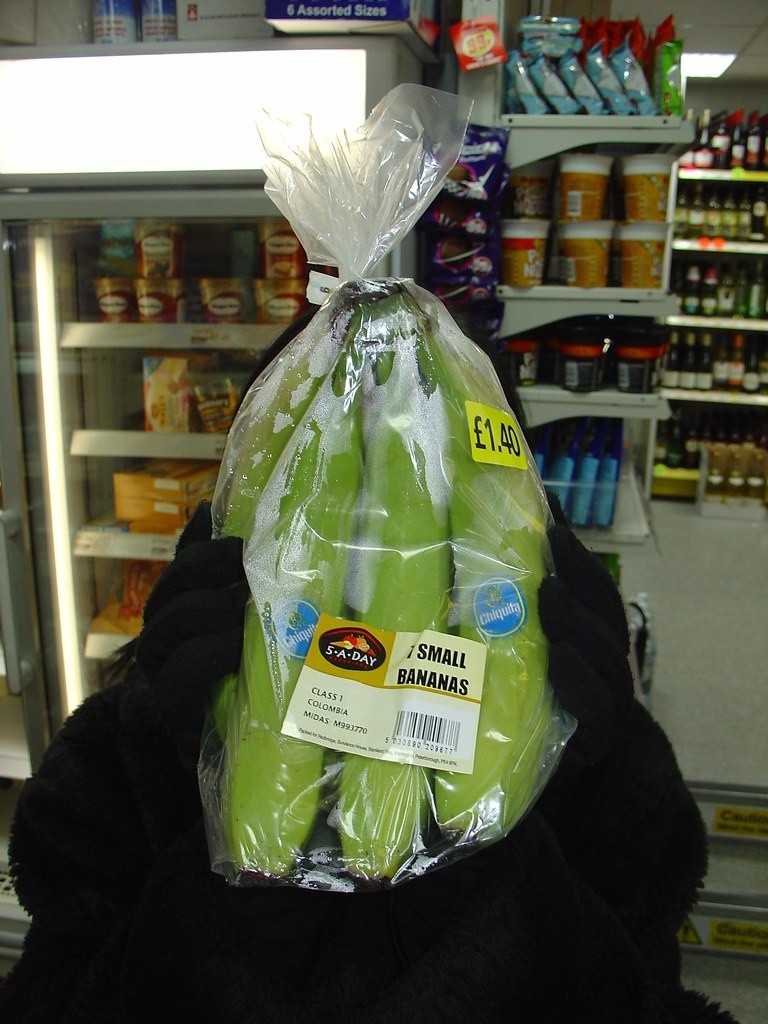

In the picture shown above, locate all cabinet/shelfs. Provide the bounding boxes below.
[0,192,394,783]
[493,112,768,558]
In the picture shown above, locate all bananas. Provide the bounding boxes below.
[204,281,553,886]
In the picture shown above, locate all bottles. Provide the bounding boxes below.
[648,108,768,509]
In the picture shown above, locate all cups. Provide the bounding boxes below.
[492,146,673,393]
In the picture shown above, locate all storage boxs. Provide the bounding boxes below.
[264,0,443,64]
[143,352,220,433]
[114,457,221,534]
[174,0,275,40]
